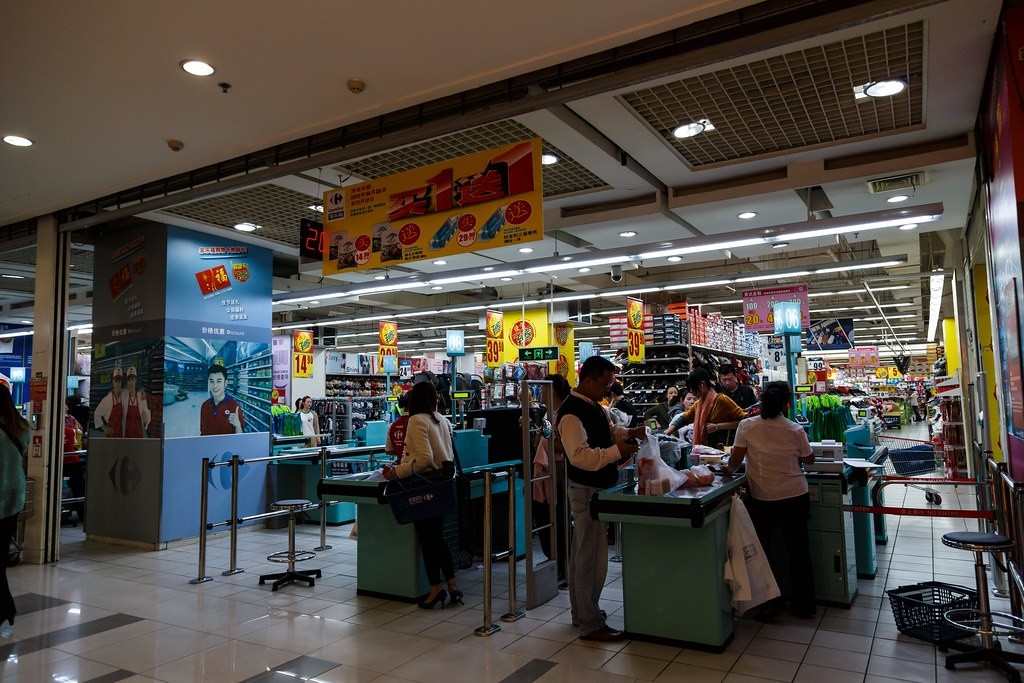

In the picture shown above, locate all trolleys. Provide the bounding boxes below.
[876,433,945,505]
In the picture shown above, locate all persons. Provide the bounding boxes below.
[921,389,933,420]
[60,398,87,534]
[94,367,152,439]
[556,355,647,642]
[294,396,321,448]
[383,381,464,610]
[0,381,31,627]
[361,355,370,374]
[443,361,449,374]
[721,380,821,616]
[533,374,571,592]
[643,385,698,444]
[714,363,756,416]
[200,364,244,436]
[516,385,538,408]
[908,388,922,421]
[663,367,749,449]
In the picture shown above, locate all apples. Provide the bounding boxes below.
[684,471,714,486]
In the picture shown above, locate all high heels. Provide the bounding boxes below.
[451,590,465,606]
[418,590,447,610]
[0,610,16,626]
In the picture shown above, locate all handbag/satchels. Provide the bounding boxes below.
[457,477,472,509]
[635,428,688,496]
[76,352,91,375]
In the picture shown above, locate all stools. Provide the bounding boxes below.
[259,500,322,590]
[940,532,1024,683]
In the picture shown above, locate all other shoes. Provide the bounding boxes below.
[579,624,624,642]
[573,609,607,627]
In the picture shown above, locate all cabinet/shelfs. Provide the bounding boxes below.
[326,346,975,536]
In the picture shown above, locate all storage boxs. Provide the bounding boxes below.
[609,302,708,349]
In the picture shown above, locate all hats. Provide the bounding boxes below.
[127,367,137,378]
[113,367,123,378]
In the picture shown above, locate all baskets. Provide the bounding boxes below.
[383,460,456,525]
[884,578,981,644]
